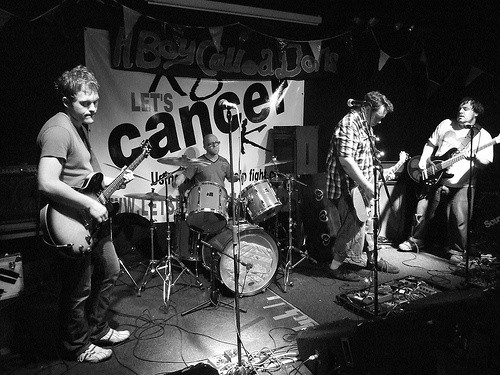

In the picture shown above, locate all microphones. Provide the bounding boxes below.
[464,123,474,132]
[218,99,237,108]
[347,99,371,107]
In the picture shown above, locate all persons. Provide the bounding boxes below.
[183,134,238,244]
[397,98,495,263]
[327,90,401,283]
[35,63,131,362]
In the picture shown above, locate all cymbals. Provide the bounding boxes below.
[258,159,293,168]
[123,191,177,202]
[156,156,212,167]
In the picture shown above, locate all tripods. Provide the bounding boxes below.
[119,110,485,375]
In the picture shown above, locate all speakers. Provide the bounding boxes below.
[297,319,423,375]
[274,125,318,175]
[403,285,500,375]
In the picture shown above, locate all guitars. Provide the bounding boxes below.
[39,140,153,259]
[420,134,500,187]
[348,151,409,224]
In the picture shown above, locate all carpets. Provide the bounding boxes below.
[267,245,457,325]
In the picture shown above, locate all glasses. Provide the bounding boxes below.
[205,141,220,148]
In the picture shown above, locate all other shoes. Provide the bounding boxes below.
[399,238,425,251]
[449,254,464,264]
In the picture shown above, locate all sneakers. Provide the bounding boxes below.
[77,344,113,363]
[100,328,131,343]
[367,257,400,274]
[328,264,360,282]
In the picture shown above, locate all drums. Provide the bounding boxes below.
[202,216,279,295]
[236,179,283,222]
[170,208,202,261]
[183,181,229,233]
